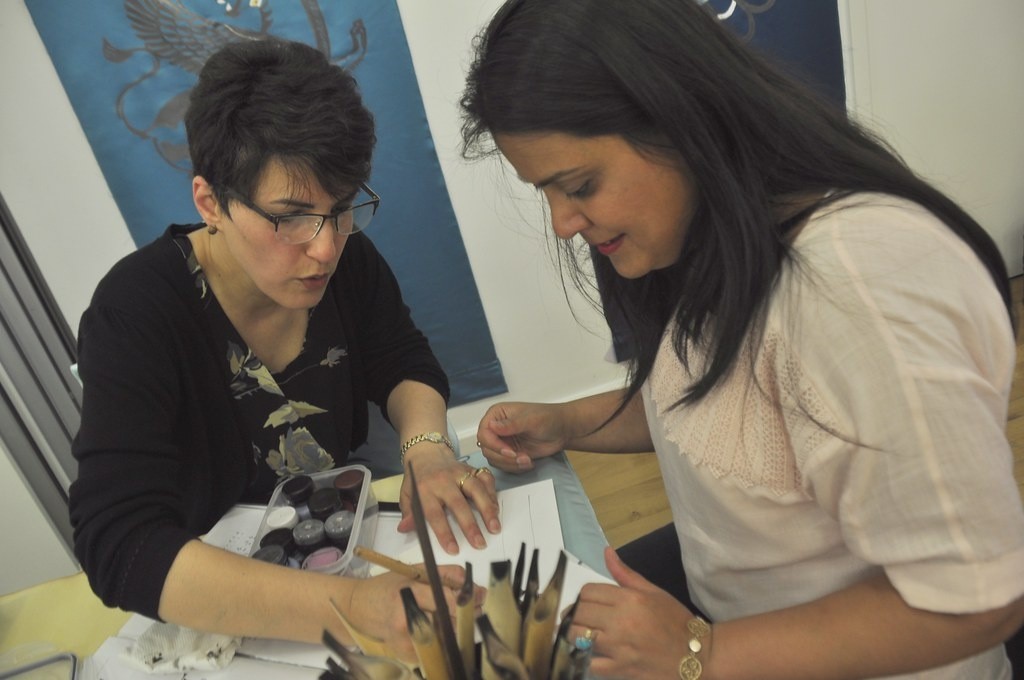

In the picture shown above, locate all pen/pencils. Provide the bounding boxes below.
[306,546,599,680]
[355,546,473,596]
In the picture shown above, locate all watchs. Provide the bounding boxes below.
[399,433,453,465]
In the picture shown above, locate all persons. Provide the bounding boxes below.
[474,0,1024,680]
[67,38,502,663]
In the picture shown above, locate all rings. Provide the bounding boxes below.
[474,467,492,479]
[459,473,476,496]
[576,629,593,651]
[475,441,482,447]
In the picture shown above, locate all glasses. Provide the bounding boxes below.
[222,185,381,246]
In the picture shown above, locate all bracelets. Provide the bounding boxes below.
[678,614,712,680]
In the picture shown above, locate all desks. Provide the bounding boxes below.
[0,441,619,680]
